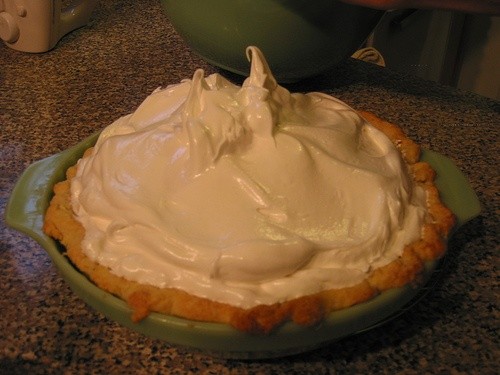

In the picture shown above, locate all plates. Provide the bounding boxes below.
[4,131,481,359]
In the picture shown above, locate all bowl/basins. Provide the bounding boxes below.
[159,0,387,84]
